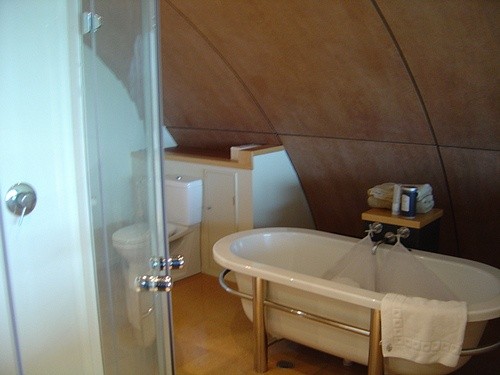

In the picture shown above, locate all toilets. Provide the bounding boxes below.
[112,175,203,287]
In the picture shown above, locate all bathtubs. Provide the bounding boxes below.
[213,227,496,375]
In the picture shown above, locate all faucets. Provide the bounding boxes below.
[371,233,396,255]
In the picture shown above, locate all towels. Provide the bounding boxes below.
[381,293,467,368]
[367,182,435,214]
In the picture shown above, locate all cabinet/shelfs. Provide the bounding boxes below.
[134,142,315,285]
